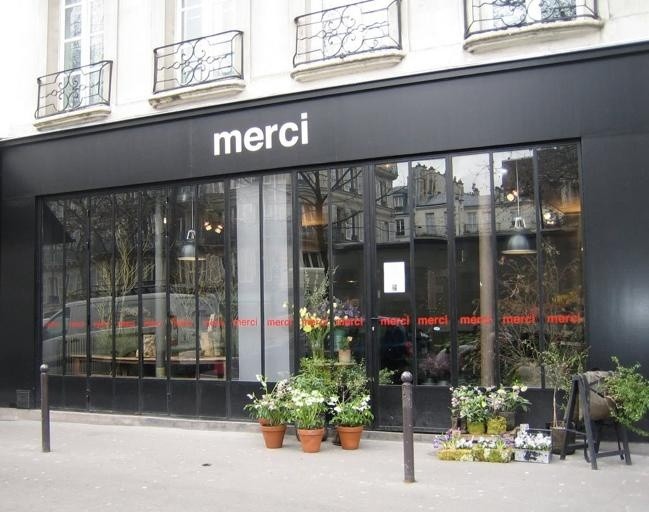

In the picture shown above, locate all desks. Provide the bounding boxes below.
[68,354,240,378]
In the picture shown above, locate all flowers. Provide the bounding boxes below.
[434,381,552,463]
[243,265,378,427]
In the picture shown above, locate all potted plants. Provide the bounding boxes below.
[520,340,592,454]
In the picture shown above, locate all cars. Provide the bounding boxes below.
[331,315,443,386]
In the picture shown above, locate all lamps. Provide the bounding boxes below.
[502,151,538,254]
[178,184,206,261]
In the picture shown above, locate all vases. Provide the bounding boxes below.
[260,424,363,452]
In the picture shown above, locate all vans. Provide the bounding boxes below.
[42,290,227,380]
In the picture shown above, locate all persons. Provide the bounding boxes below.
[382,312,412,381]
